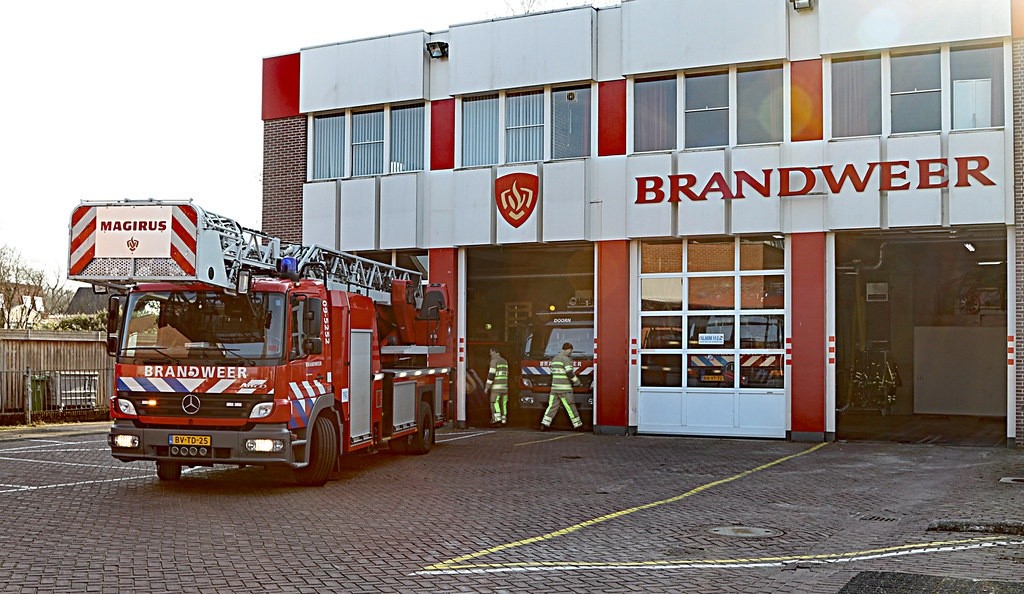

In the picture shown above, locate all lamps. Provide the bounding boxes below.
[789,0,810,11]
[426,42,448,59]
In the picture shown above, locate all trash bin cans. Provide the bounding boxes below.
[23,374,47,411]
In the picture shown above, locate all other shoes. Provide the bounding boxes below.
[490,416,502,423]
[541,424,547,431]
[502,419,507,423]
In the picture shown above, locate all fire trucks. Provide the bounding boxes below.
[519,297,594,421]
[66,198,453,485]
[641,315,785,388]
[466,340,519,415]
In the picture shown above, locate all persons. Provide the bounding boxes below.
[169,314,208,342]
[539,342,588,433]
[484,346,509,429]
